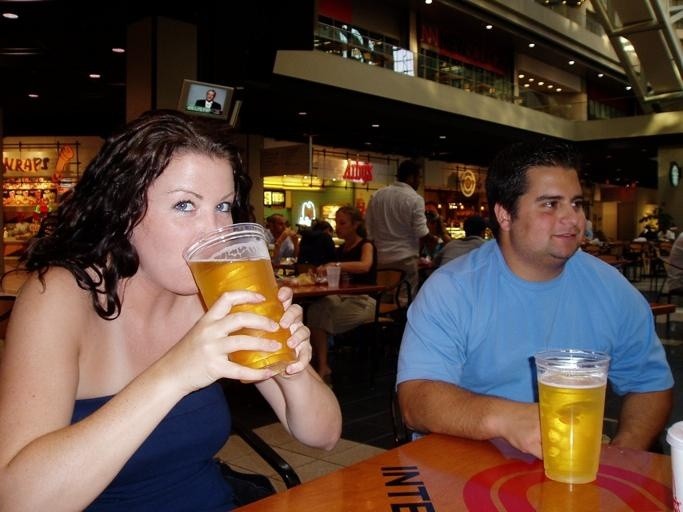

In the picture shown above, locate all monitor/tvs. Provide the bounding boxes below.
[178,79,235,120]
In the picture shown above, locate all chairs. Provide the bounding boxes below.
[377,267,413,316]
[581,237,681,294]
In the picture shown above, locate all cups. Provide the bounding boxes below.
[533,348,612,486]
[666,420,683,504]
[326,267,341,289]
[183,223,299,369]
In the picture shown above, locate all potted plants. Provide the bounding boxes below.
[638,200,673,239]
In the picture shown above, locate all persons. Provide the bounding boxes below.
[2,113,338,512]
[308,206,378,383]
[657,215,674,253]
[195,90,221,110]
[297,221,339,266]
[437,215,486,263]
[394,148,674,462]
[263,214,301,269]
[365,160,427,310]
